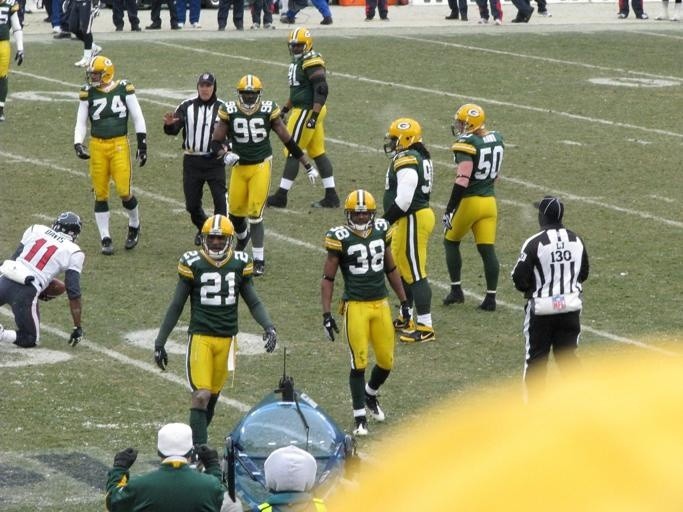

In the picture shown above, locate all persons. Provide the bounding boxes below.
[18,0,28,28]
[164,73,239,245]
[63,0,102,67]
[654,1,681,22]
[107,423,225,512]
[442,103,504,310]
[0,211,85,349]
[176,1,202,29]
[477,0,503,24]
[536,1,551,16]
[110,1,142,32]
[155,214,278,447]
[366,0,390,22]
[322,189,412,437]
[513,195,590,400]
[383,118,435,343]
[218,0,247,30]
[73,56,148,255]
[446,0,469,21]
[209,75,320,277]
[41,0,75,39]
[280,0,332,24]
[266,29,340,209]
[510,0,534,23]
[147,1,180,31]
[616,0,648,20]
[251,0,276,31]
[1,1,25,121]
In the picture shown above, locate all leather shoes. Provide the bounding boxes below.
[511,16,524,23]
[446,13,458,20]
[524,6,534,24]
[462,16,468,20]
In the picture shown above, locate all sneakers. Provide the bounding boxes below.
[178,23,185,28]
[54,32,72,39]
[495,19,500,25]
[237,27,243,30]
[235,233,251,251]
[381,16,389,22]
[478,17,489,23]
[267,194,286,207]
[171,25,182,29]
[93,46,102,56]
[195,230,202,247]
[125,223,140,248]
[310,196,340,207]
[398,322,435,343]
[250,23,260,29]
[393,317,415,333]
[1,115,4,120]
[252,259,265,275]
[73,56,91,66]
[353,417,368,436]
[146,24,162,29]
[477,296,497,312]
[192,22,201,27]
[442,290,465,304]
[131,26,143,31]
[115,27,122,30]
[218,27,224,31]
[264,23,277,30]
[363,18,372,21]
[101,236,114,254]
[320,16,333,24]
[538,11,551,18]
[366,401,387,422]
[280,16,296,25]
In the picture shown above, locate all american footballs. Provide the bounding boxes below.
[44,278,66,297]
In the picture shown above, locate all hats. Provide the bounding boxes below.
[197,73,216,93]
[156,423,195,456]
[264,446,317,491]
[533,194,563,222]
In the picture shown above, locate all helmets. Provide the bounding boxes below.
[85,55,114,86]
[344,189,378,231]
[235,74,263,111]
[384,117,423,158]
[450,104,486,135]
[52,211,82,237]
[286,27,312,60]
[202,214,235,258]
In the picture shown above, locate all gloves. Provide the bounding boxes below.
[402,301,410,328]
[196,445,221,467]
[113,448,137,468]
[223,150,241,166]
[305,117,315,128]
[262,326,278,353]
[68,327,84,347]
[305,165,318,181]
[75,143,89,159]
[441,208,452,228]
[322,311,340,340]
[136,146,147,166]
[154,347,168,370]
[14,51,25,65]
